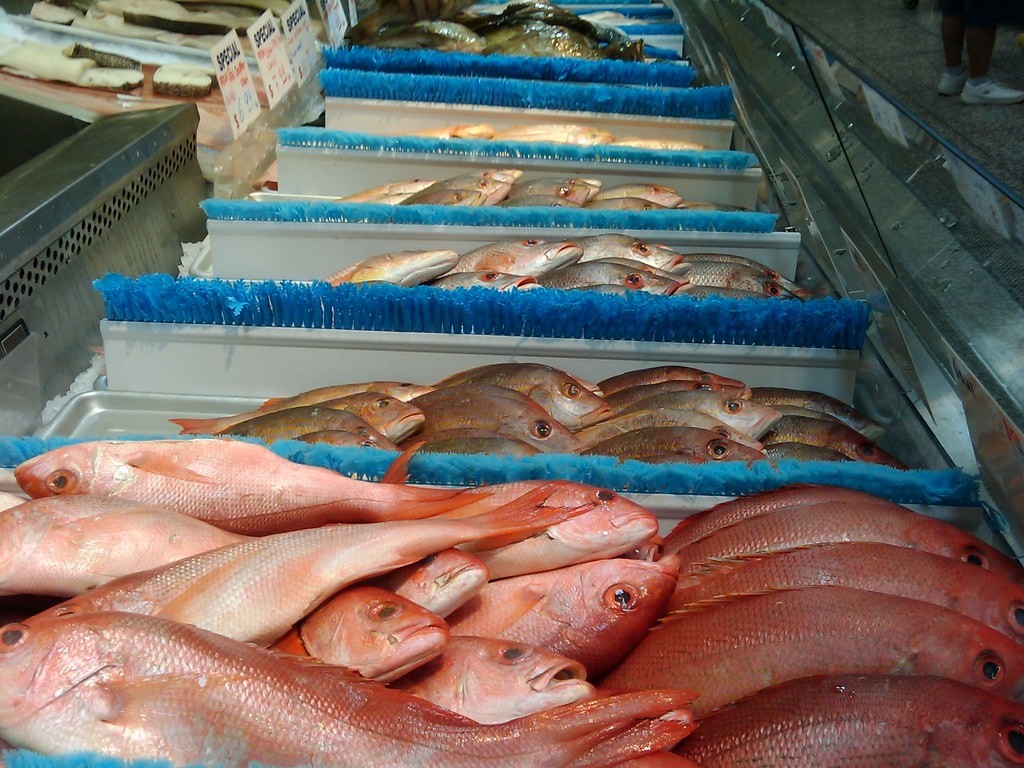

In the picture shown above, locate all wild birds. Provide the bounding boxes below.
[1,0,1024,768]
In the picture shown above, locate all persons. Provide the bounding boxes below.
[936,0,1024,105]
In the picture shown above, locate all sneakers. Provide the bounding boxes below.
[960,79,1024,104]
[939,74,968,95]
